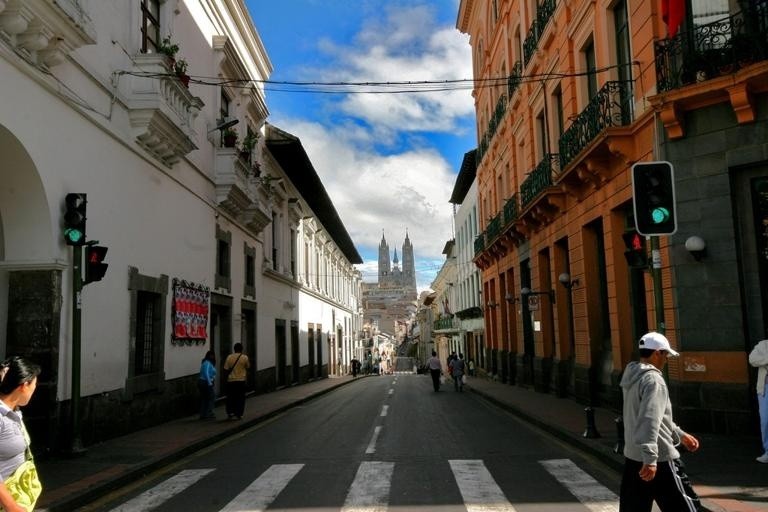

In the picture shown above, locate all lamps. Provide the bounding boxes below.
[558,274,578,288]
[684,237,706,263]
[486,287,556,310]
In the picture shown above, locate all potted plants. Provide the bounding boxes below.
[161,36,190,88]
[224,127,262,177]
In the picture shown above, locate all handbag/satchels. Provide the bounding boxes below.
[461,374,467,384]
[439,373,446,385]
[0,415,42,512]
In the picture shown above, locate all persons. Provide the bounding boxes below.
[423,349,477,394]
[618,330,708,511]
[0,358,11,383]
[350,354,359,378]
[223,342,251,421]
[196,350,217,422]
[1,354,42,512]
[748,337,768,464]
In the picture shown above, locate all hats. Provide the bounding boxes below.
[638,332,681,358]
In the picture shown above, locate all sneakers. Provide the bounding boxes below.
[756,452,768,463]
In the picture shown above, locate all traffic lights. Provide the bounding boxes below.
[83,242,108,284]
[632,162,678,237]
[61,192,87,247]
[622,230,647,269]
